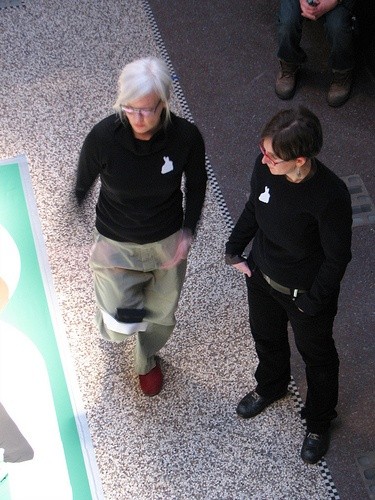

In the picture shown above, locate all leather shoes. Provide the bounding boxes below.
[236,384,289,418]
[301,418,330,464]
[276,57,299,99]
[327,68,353,106]
[139,365,163,395]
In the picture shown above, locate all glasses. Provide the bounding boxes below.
[257,139,301,167]
[118,97,164,116]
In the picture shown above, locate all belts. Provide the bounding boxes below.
[259,273,310,299]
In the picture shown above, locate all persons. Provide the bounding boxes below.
[276,0,356,107]
[225,107,352,463]
[74,56,209,397]
